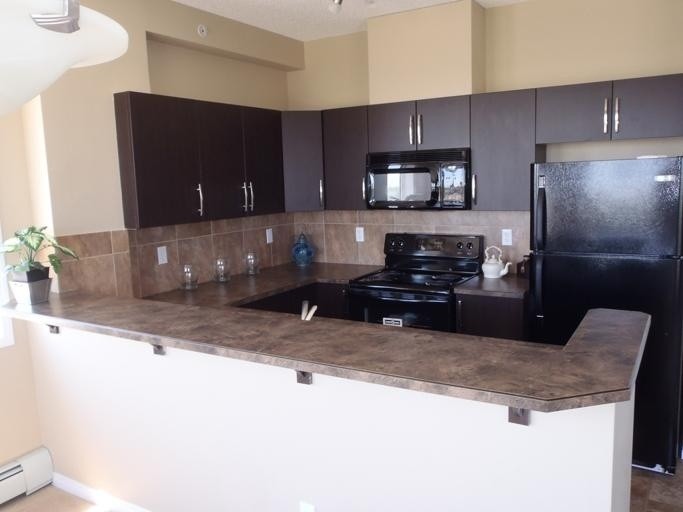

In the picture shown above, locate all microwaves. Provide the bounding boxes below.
[367,162,466,208]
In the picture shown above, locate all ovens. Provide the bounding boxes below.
[348,290,455,333]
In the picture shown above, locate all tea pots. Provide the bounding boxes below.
[481,246,512,278]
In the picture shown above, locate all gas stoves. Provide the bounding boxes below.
[349,268,477,293]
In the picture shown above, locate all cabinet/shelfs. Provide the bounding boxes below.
[471,89,536,210]
[281,105,368,213]
[114,91,207,230]
[202,73,285,229]
[455,293,529,341]
[237,283,349,319]
[536,73,683,144]
[370,93,470,152]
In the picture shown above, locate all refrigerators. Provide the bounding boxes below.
[529,155,683,476]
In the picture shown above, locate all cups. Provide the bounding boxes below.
[183,264,198,289]
[246,253,259,275]
[216,258,231,281]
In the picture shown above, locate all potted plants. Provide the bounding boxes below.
[1,226,80,305]
